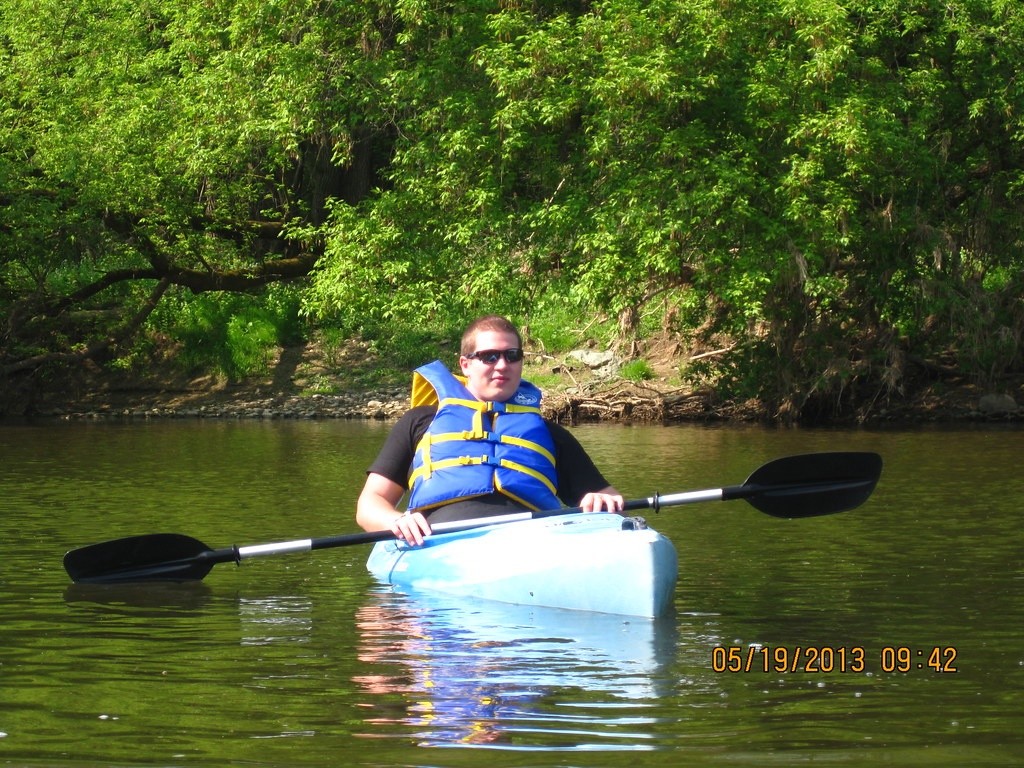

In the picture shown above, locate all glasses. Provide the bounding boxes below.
[464,348,523,365]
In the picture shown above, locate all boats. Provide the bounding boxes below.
[366,581,680,702]
[368,511,681,622]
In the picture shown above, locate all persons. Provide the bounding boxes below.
[355,316,623,546]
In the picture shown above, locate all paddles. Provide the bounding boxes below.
[62,451,884,582]
